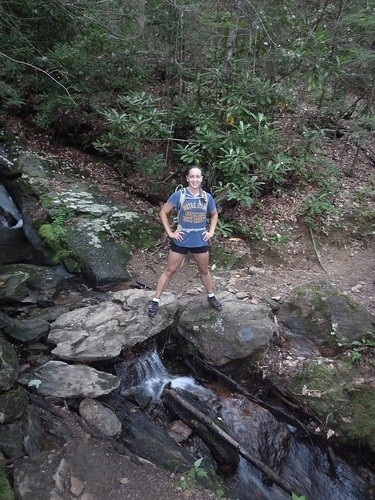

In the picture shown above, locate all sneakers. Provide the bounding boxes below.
[207,296,222,311]
[147,300,158,317]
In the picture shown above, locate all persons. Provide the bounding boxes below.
[147,166,222,317]
